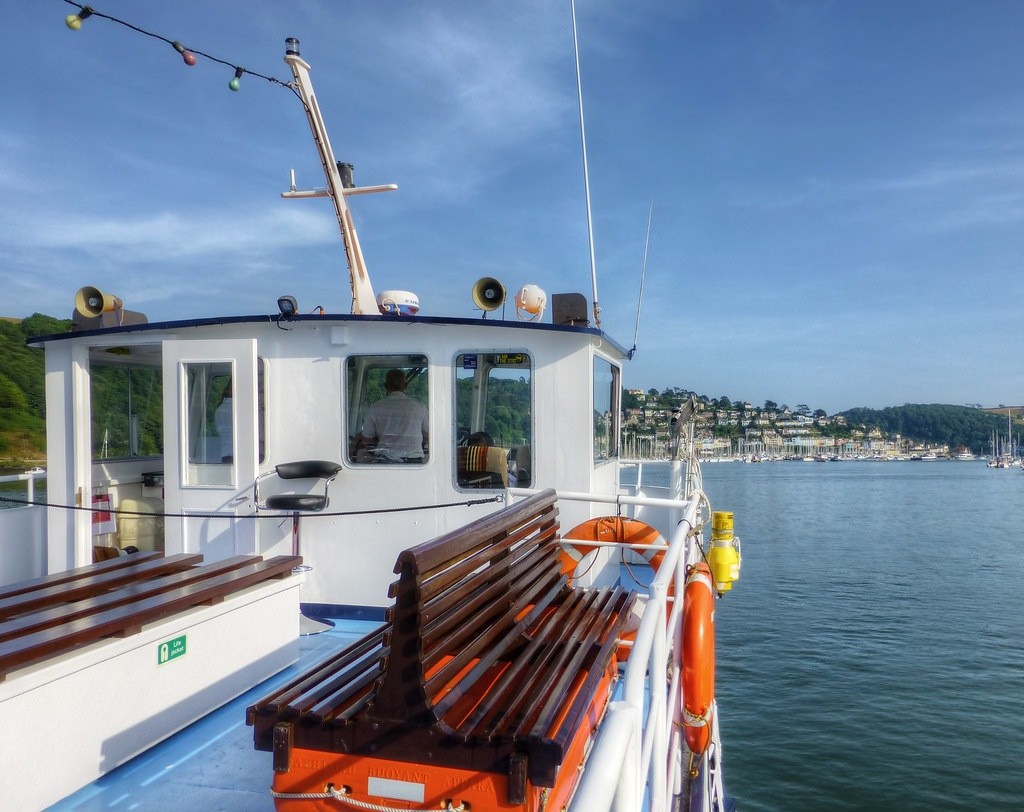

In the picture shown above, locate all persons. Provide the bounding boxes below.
[362,368,429,464]
[467,431,517,488]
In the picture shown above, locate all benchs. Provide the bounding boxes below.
[409,488,637,803]
[245,492,538,772]
[0,554,304,684]
[0,552,204,621]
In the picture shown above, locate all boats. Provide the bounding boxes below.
[955,454,975,459]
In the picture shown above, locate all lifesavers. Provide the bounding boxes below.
[550,513,679,666]
[675,559,719,757]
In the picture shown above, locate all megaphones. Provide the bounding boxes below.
[472,277,506,310]
[75,285,123,318]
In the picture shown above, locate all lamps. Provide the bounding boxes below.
[277,294,298,314]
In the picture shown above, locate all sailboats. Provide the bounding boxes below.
[988,408,1024,470]
[622,436,942,462]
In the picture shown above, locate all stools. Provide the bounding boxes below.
[253,460,343,637]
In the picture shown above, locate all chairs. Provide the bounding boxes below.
[457,445,509,488]
